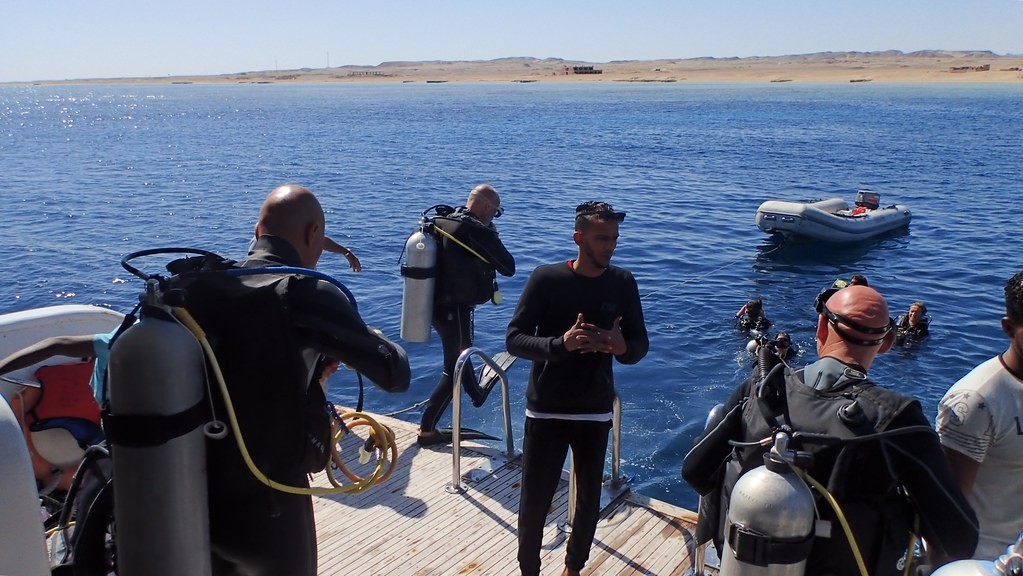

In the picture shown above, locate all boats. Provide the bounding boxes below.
[755,189,912,244]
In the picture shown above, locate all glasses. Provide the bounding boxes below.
[493,207,505,218]
[575,210,626,224]
[813,285,840,312]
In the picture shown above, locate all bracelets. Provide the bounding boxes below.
[344,249,350,257]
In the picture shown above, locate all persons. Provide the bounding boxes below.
[417,185,517,446]
[895,300,928,345]
[184,183,411,575]
[772,332,795,359]
[247,232,361,272]
[922,271,1023,562]
[850,275,869,286]
[735,296,767,322]
[505,200,649,576]
[681,285,980,576]
